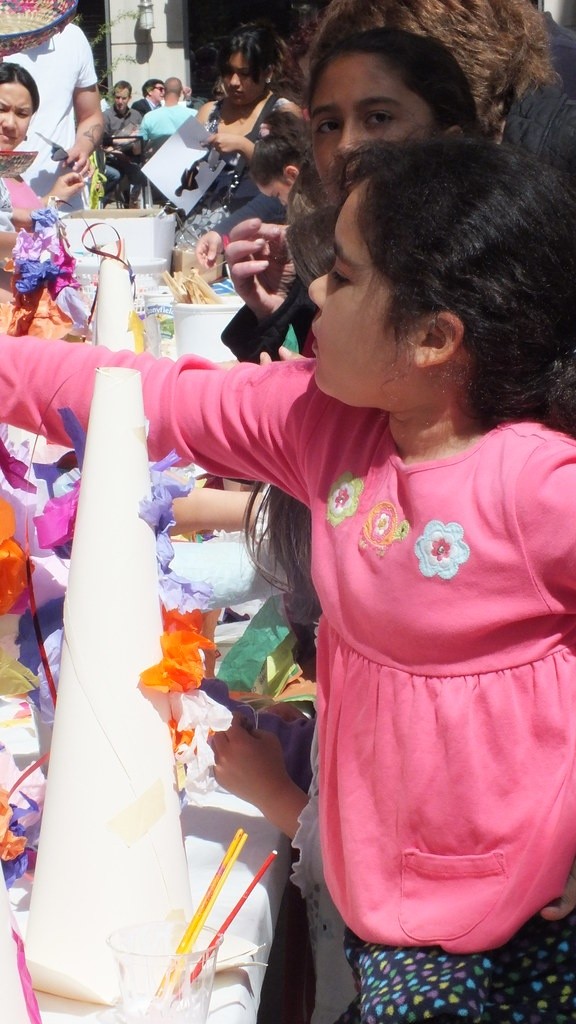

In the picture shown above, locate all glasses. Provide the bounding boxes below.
[152,86,165,92]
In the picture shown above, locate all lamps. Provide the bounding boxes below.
[138,1,155,31]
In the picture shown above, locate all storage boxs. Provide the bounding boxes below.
[171,248,223,284]
[61,207,175,287]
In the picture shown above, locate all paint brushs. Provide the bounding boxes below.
[141,828,279,1024]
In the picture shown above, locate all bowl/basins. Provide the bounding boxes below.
[172,297,247,364]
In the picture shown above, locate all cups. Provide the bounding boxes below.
[105,921,225,1024]
[142,292,175,358]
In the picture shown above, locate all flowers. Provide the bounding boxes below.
[0,203,235,891]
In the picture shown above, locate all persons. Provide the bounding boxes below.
[0,0,576,1024]
[0,132,576,1024]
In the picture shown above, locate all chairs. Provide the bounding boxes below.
[105,134,147,209]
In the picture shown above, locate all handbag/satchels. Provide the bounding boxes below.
[174,204,231,248]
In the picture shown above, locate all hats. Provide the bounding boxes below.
[0,0,78,57]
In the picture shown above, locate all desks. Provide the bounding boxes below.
[0,541,291,1024]
